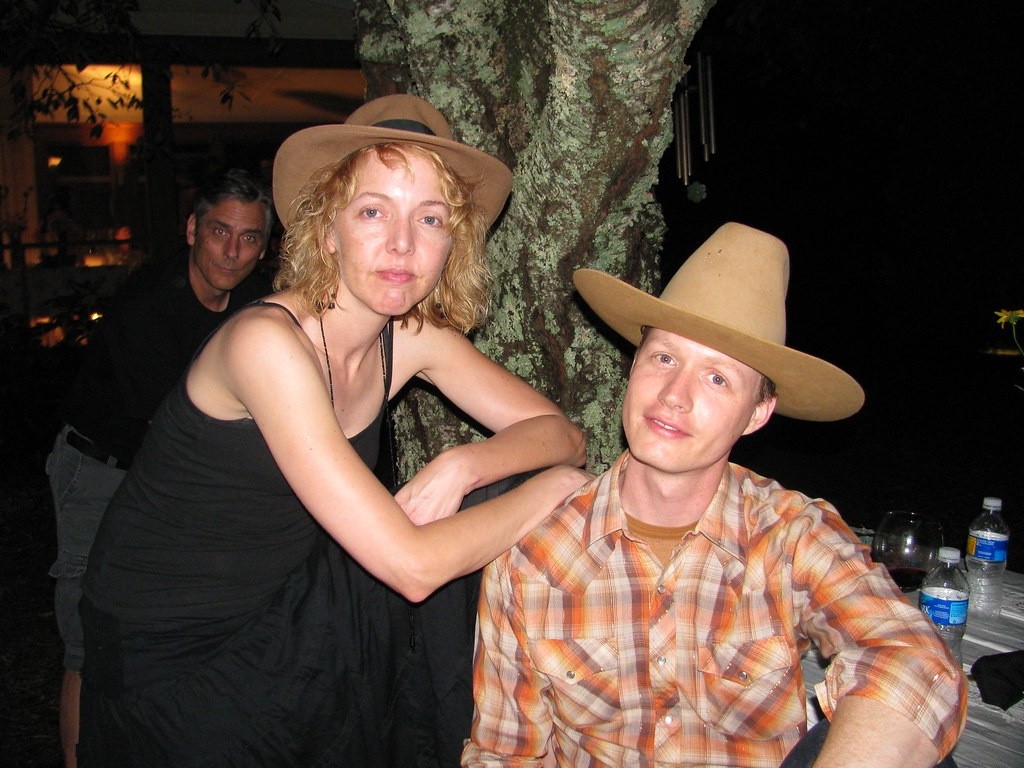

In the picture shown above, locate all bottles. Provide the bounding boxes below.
[965,497,1009,618]
[916,548,970,670]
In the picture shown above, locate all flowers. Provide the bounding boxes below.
[995,309,1024,354]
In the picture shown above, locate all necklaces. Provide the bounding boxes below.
[317,298,396,496]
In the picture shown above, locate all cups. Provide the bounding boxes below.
[871,511,943,593]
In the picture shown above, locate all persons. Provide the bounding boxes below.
[45,171,272,768]
[78,95,593,768]
[460,223,970,768]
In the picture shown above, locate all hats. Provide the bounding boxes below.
[270,92,525,243]
[571,219,866,422]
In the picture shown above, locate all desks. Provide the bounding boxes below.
[801,566,1024,768]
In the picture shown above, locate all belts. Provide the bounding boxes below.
[64,431,133,470]
